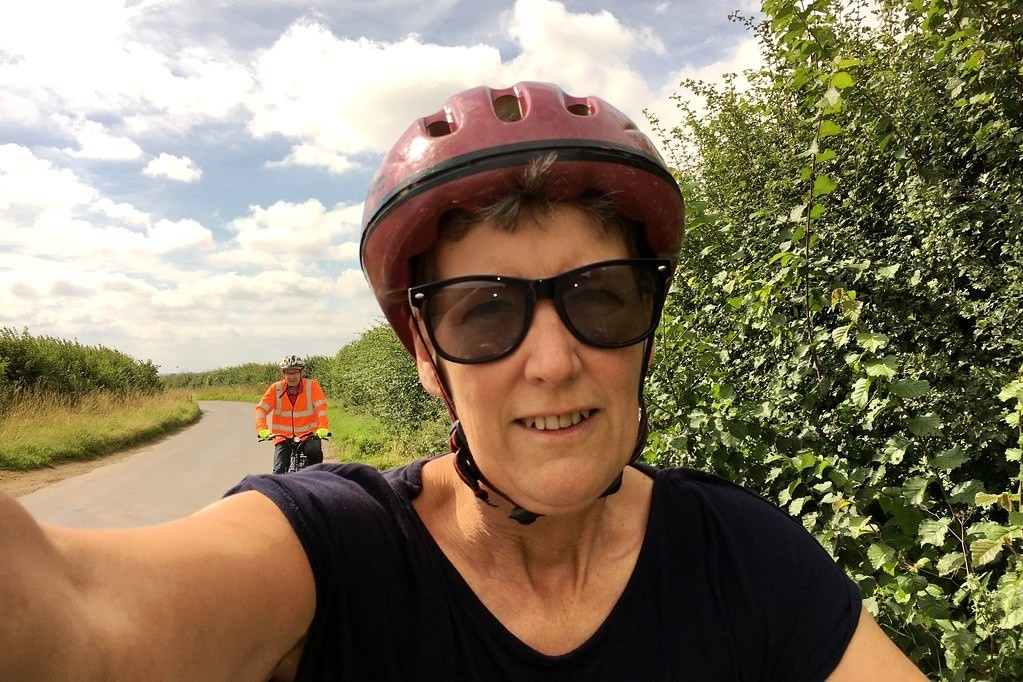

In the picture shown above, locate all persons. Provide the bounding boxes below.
[255,354,330,475]
[0,80,930,682]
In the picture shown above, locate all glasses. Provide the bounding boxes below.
[284,372,302,376]
[410,258,675,364]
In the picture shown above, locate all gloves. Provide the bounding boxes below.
[314,428,330,439]
[259,430,273,441]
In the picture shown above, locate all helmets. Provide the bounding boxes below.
[280,355,305,370]
[359,82,686,359]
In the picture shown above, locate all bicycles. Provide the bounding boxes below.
[258,430,332,474]
